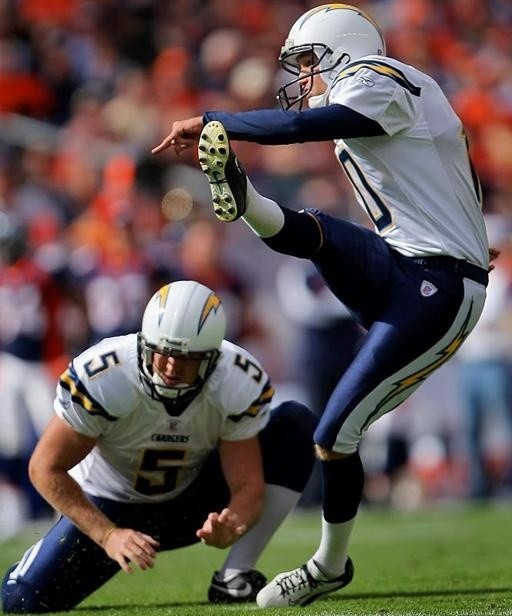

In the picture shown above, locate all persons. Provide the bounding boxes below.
[2,0,511,499]
[152,0,490,610]
[2,280,320,614]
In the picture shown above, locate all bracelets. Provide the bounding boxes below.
[101,526,117,550]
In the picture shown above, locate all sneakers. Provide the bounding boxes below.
[207,556,354,610]
[197,121,246,223]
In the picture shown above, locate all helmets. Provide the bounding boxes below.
[276,5,386,113]
[137,280,225,404]
